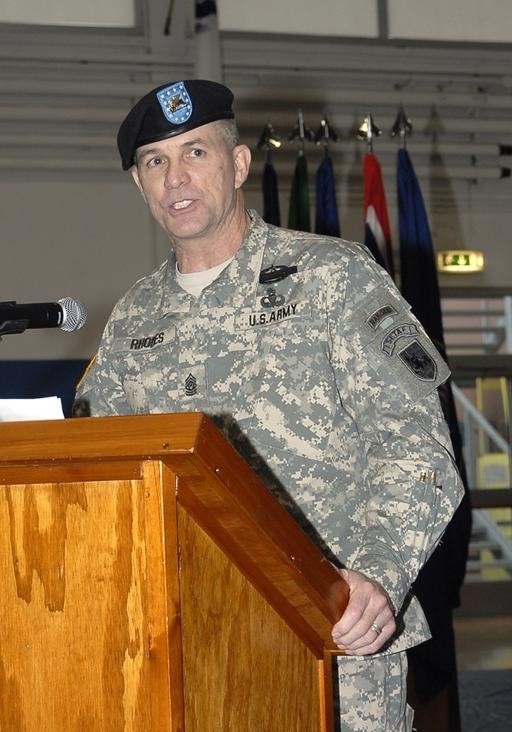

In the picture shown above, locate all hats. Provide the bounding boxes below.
[117,79,233,170]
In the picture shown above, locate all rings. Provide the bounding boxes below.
[371,623,381,635]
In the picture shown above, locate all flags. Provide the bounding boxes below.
[286,155,309,232]
[362,153,396,281]
[313,151,342,237]
[394,148,460,441]
[260,161,282,226]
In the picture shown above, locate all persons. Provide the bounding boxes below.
[72,80,466,732]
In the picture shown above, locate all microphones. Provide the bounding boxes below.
[0,295,89,336]
